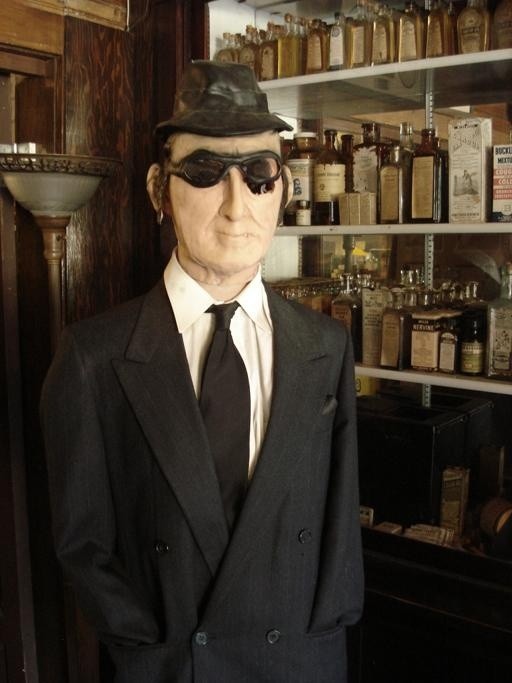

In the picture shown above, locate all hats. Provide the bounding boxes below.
[152,60,293,137]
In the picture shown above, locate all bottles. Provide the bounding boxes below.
[276,121,447,225]
[277,246,509,374]
[215,0,512,83]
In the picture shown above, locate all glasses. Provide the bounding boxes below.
[168,149,284,187]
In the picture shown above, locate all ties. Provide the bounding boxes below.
[199,300,250,535]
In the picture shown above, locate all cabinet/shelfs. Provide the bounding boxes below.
[200,0,512,680]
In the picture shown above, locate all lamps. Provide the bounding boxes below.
[2,152,126,344]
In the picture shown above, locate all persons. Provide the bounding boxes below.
[37,56,365,682]
